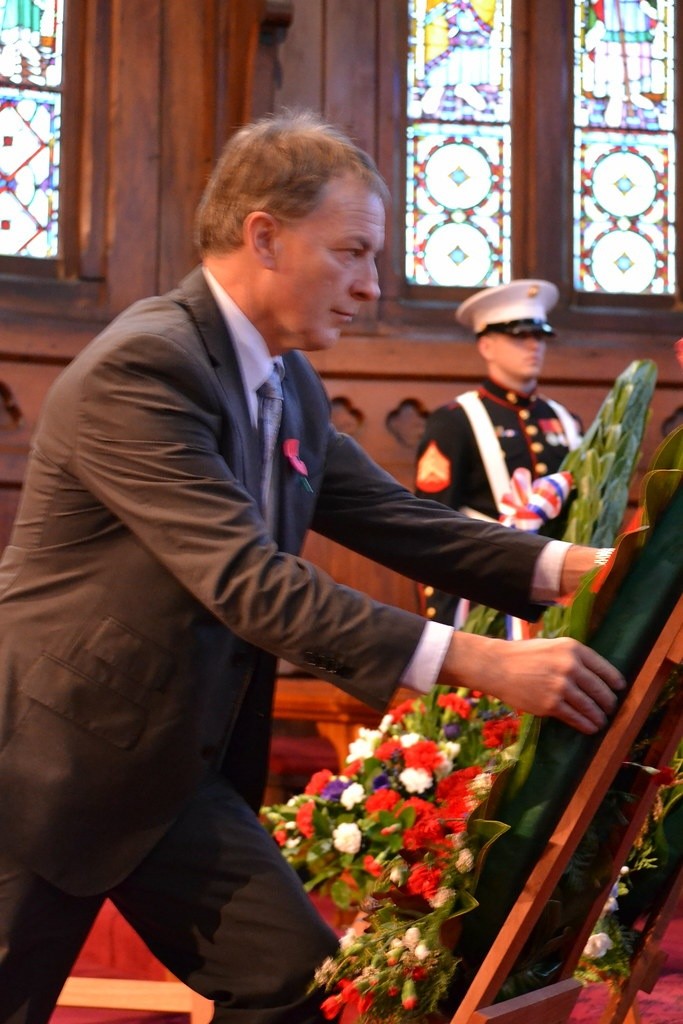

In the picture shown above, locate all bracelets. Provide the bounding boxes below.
[595,547,614,573]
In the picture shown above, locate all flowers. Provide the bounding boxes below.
[260,685,632,1023]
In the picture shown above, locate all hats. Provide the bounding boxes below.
[454,278,561,337]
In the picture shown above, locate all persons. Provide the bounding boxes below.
[0,109,627,1023]
[415,277,586,627]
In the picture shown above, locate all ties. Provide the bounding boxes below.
[256,364,284,532]
